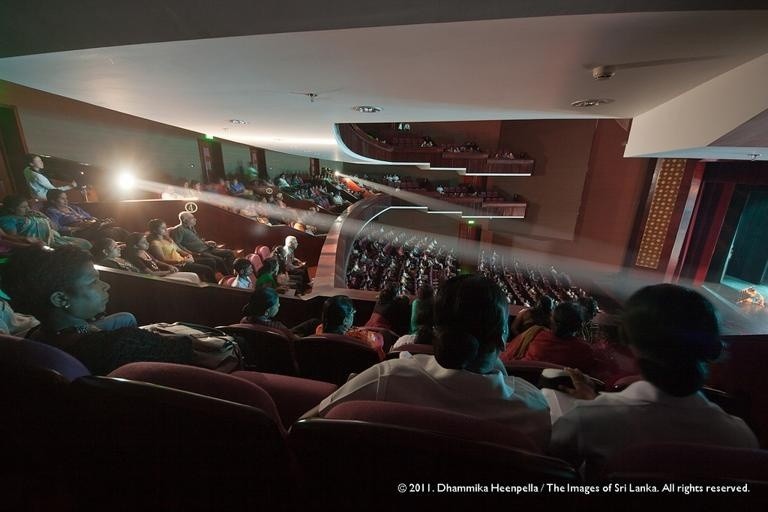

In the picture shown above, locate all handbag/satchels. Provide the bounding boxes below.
[136,321,245,373]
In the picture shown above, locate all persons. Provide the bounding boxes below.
[550,284,760,458]
[289,274,550,440]
[0,135,600,384]
[734,284,767,308]
